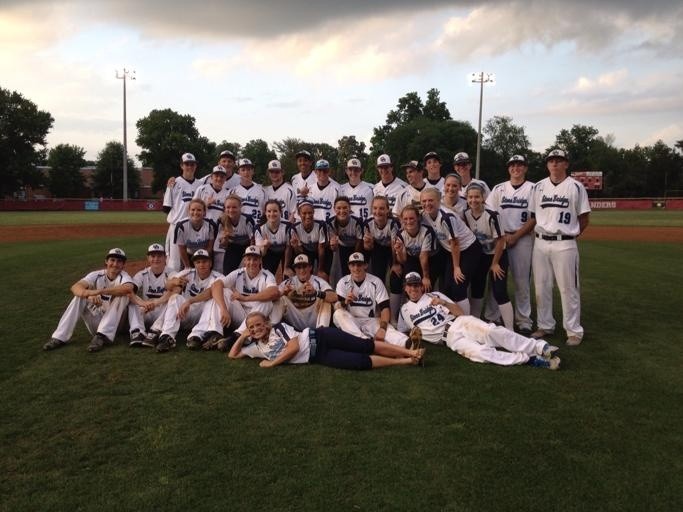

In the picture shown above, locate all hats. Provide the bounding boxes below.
[293,254,310,267]
[347,252,366,264]
[182,154,197,165]
[243,245,260,258]
[107,247,127,260]
[213,152,470,172]
[145,243,164,254]
[406,272,421,284]
[191,249,209,261]
[506,154,526,165]
[545,150,567,161]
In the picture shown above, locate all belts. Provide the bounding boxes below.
[535,233,573,241]
[309,329,317,362]
[443,319,455,345]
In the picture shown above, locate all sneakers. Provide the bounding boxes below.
[523,330,584,369]
[409,327,423,349]
[43,332,234,350]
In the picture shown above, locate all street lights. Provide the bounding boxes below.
[114,66,136,203]
[466,72,492,183]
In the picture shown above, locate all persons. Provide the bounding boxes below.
[41,246,140,352]
[162,151,560,371]
[530,151,591,346]
[126,242,184,350]
